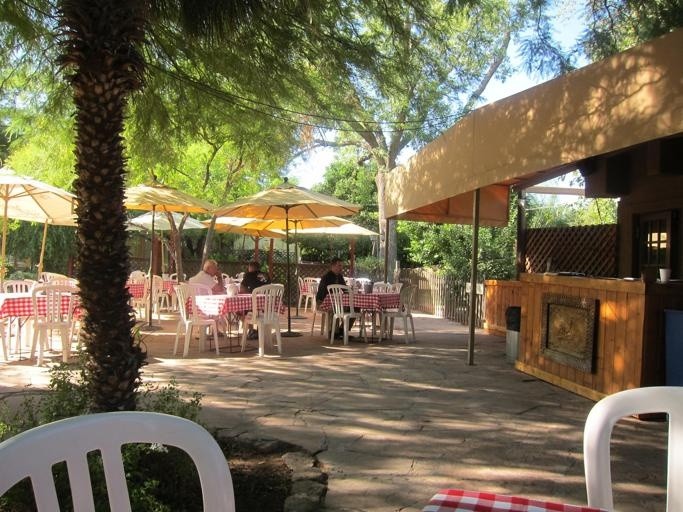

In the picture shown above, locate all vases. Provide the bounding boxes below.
[658,267,671,281]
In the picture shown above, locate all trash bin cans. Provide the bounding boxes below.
[505,306,521,364]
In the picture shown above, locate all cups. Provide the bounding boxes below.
[363,282,374,294]
[659,268,673,283]
[227,285,239,296]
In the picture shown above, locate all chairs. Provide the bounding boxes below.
[298,274,416,347]
[3,271,86,365]
[0,409,235,512]
[581,386,683,512]
[125,271,284,355]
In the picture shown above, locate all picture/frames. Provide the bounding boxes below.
[534,291,599,375]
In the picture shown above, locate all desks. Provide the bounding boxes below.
[421,489,618,512]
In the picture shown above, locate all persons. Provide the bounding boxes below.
[188,257,272,340]
[316,258,362,339]
